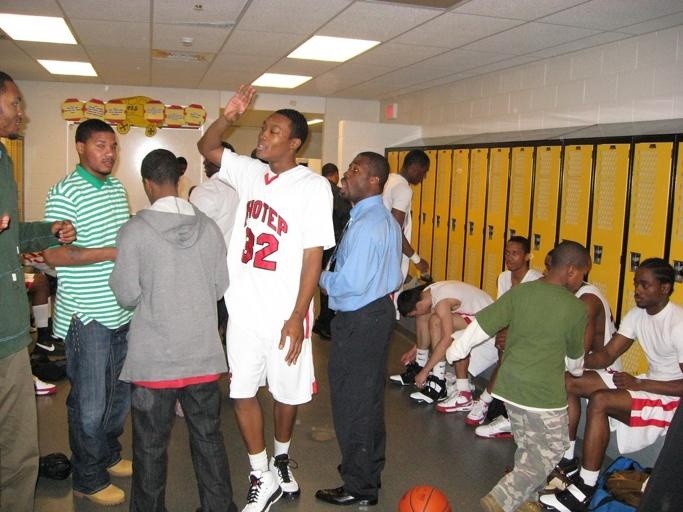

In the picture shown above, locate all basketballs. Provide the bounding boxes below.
[398,486,452,511]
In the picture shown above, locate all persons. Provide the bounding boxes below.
[0,69,39,512]
[39,117,135,508]
[319,140,431,342]
[196,82,337,511]
[109,142,240,510]
[389,279,501,406]
[439,237,683,512]
[315,150,402,509]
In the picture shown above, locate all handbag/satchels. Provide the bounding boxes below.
[602,470,651,508]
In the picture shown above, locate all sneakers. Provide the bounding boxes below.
[240,470,283,512]
[268,456,300,502]
[389,361,598,512]
[32,333,68,396]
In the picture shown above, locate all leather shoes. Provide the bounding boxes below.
[106,459,133,478]
[74,484,127,506]
[314,463,378,506]
